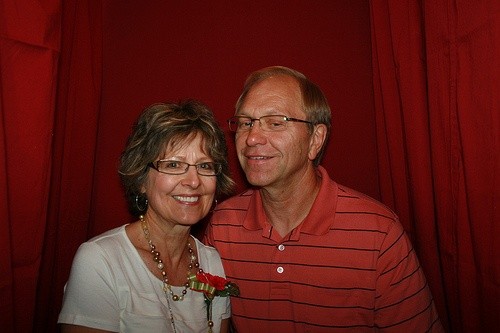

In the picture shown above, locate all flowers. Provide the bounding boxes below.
[188,272,240,321]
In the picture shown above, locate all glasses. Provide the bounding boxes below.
[226,114,314,133]
[146,160,223,177]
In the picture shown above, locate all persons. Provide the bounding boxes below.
[198,65,445,333]
[56,98,241,333]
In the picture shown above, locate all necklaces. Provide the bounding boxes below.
[139,213,213,333]
